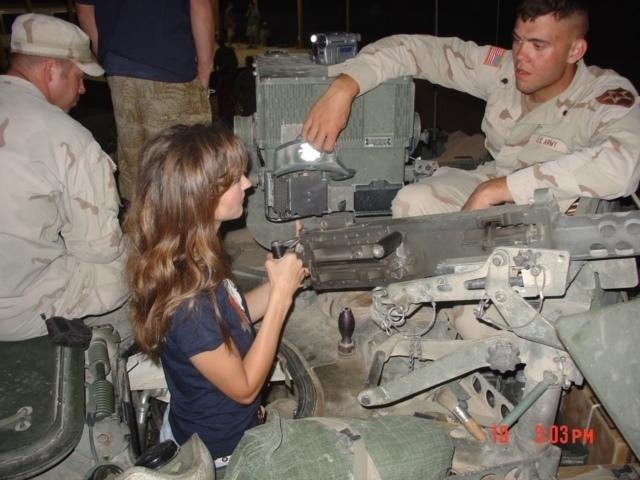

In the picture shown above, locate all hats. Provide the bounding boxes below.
[11,14,106,76]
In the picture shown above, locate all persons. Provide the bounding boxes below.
[298,0,640,221]
[76,0,215,212]
[118,124,311,471]
[0,13,140,354]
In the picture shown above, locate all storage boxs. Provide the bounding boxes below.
[248,54,417,189]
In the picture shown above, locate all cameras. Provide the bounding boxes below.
[310,32,361,66]
